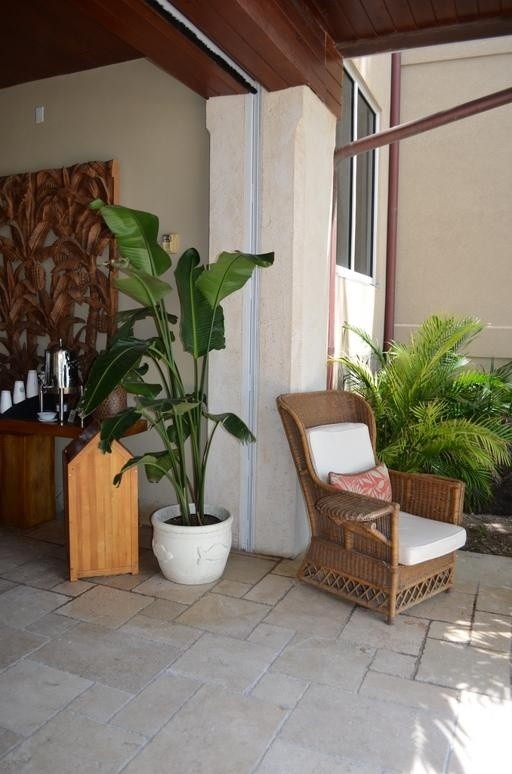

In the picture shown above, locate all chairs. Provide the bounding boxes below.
[278,384,467,623]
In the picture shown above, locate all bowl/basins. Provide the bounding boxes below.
[37,412,57,419]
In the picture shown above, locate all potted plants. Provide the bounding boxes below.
[68,194,275,588]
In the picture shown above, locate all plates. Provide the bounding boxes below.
[38,418,59,422]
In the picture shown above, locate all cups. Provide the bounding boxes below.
[0,370,39,415]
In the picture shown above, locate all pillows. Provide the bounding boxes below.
[323,463,397,521]
